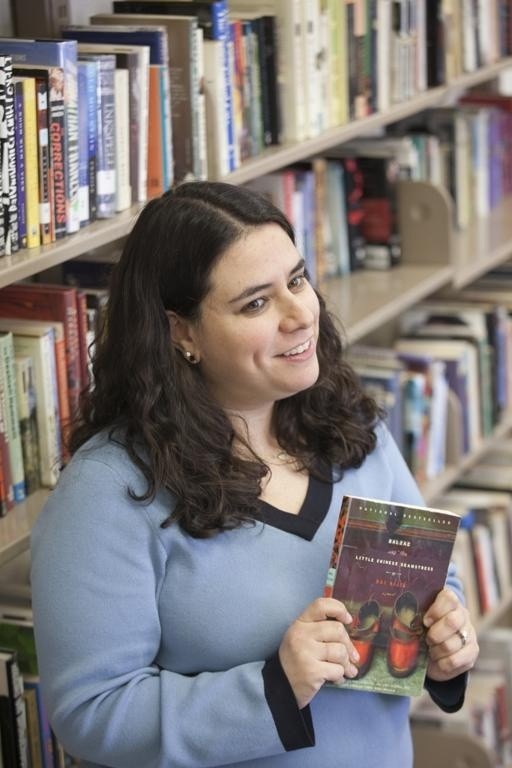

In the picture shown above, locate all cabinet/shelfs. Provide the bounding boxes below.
[1,0,512,768]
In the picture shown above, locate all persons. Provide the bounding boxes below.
[29,183,481,768]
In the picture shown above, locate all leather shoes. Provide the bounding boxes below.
[387,592,424,678]
[346,601,381,680]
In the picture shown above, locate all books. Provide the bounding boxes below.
[411,501,512,766]
[324,495,461,699]
[0,606,67,768]
[258,94,511,289]
[345,272,511,478]
[2,0,510,260]
[1,256,116,519]
